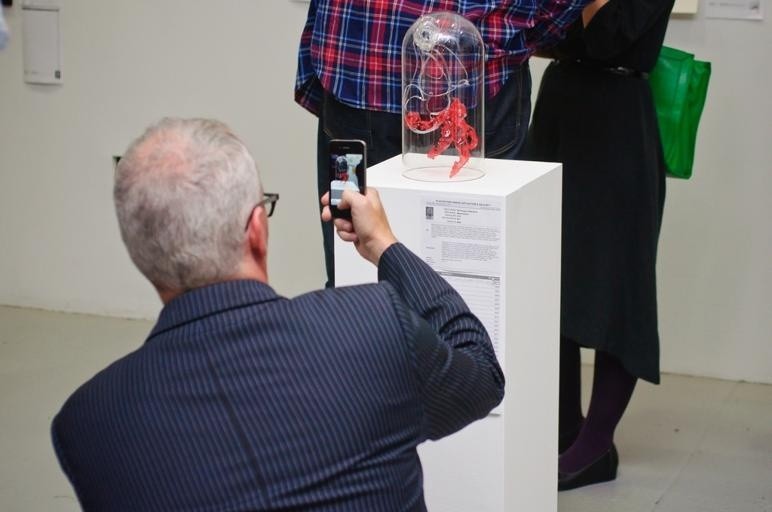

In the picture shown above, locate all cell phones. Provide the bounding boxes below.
[329,139,367,222]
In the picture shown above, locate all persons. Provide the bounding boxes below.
[526,0,677,492]
[48,114,506,512]
[291,0,584,291]
[333,154,347,182]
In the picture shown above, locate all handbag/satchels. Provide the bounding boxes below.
[648,41,712,181]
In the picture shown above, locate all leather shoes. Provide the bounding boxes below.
[559,416,618,492]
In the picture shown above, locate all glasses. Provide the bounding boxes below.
[243,190,279,232]
